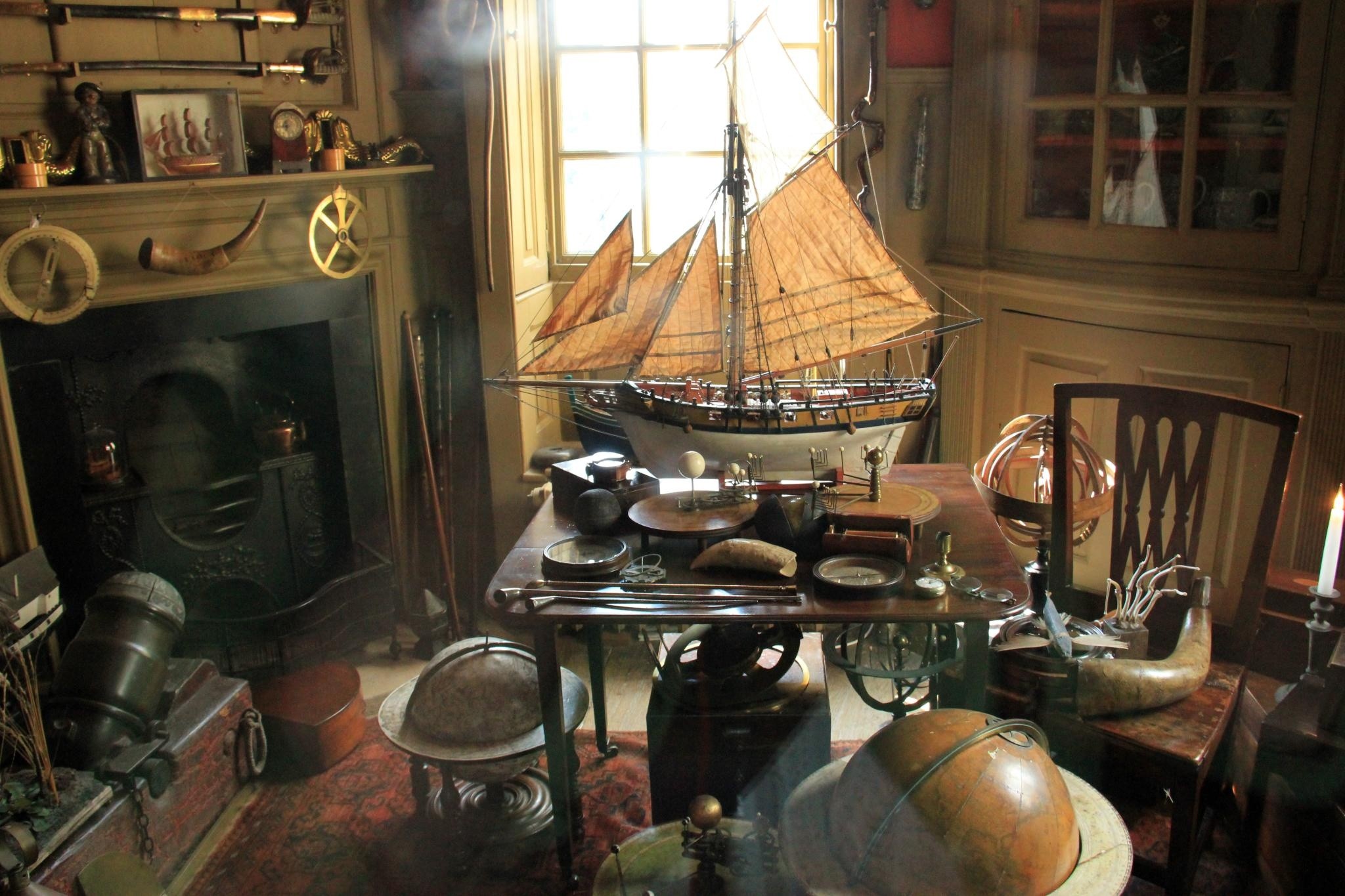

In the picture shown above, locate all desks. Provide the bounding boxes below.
[1233,671,1344,895]
[482,468,1032,895]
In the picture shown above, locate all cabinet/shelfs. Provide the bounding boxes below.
[929,0,1345,634]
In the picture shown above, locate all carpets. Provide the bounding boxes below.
[186,720,1344,896]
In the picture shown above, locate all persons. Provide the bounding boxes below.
[71,81,119,183]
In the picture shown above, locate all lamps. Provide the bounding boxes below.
[1318,481,1345,594]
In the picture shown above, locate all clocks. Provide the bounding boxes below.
[270,102,314,175]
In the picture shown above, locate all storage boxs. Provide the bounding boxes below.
[551,453,660,534]
[248,657,367,774]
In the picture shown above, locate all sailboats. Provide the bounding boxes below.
[481,10,988,485]
[146,107,228,176]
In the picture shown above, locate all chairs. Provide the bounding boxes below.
[939,382,1308,895]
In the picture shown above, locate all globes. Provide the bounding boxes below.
[776,707,1133,896]
[376,634,588,847]
